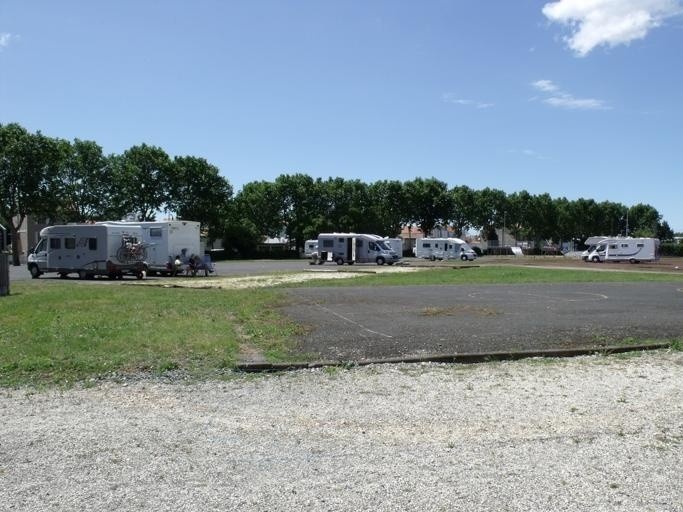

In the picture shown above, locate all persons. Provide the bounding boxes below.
[189,254,214,273]
[174,255,195,277]
[181,249,190,264]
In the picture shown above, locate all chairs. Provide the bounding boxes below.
[168,254,208,277]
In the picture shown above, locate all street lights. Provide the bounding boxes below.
[503,210,507,247]
